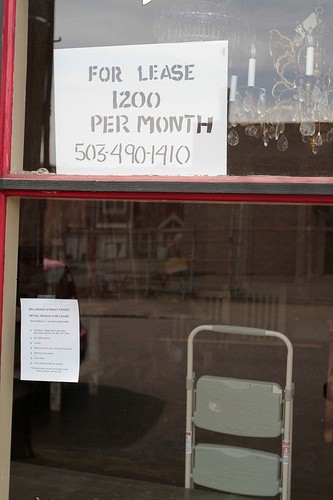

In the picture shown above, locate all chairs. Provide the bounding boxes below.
[184,324,295,500]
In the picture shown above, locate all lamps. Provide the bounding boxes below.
[149,0,332,155]
